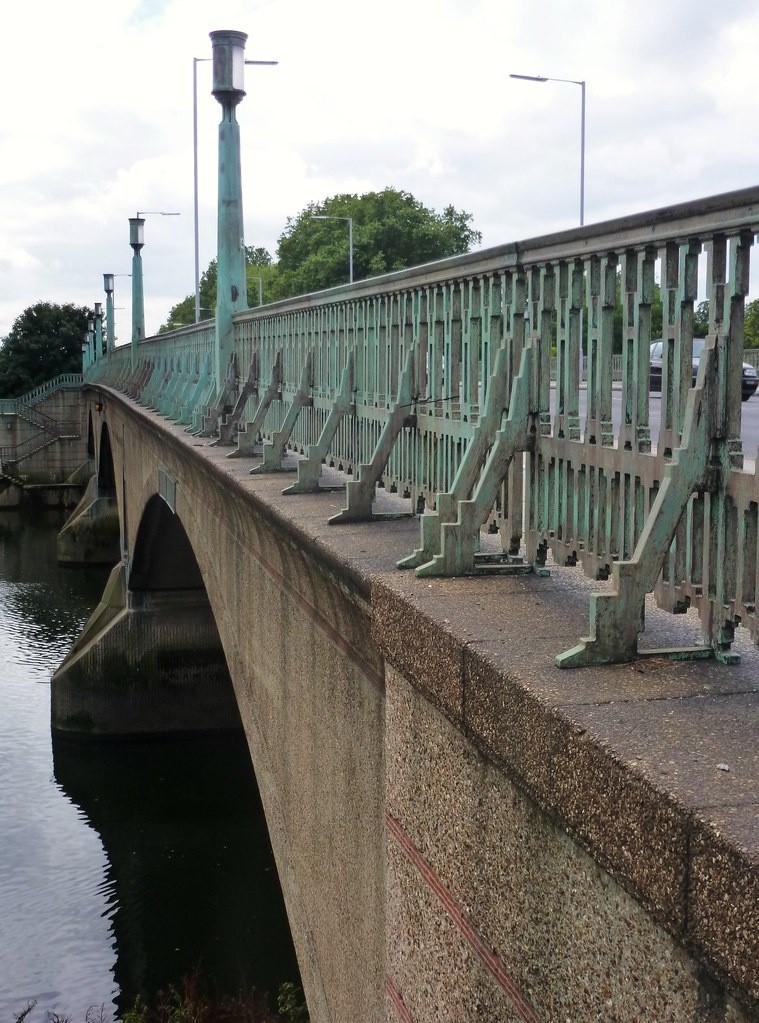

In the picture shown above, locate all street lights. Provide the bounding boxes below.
[128,211,181,341]
[310,216,353,283]
[81,273,131,367]
[194,30,278,324]
[509,72,585,388]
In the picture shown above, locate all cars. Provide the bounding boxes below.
[649,337,758,401]
